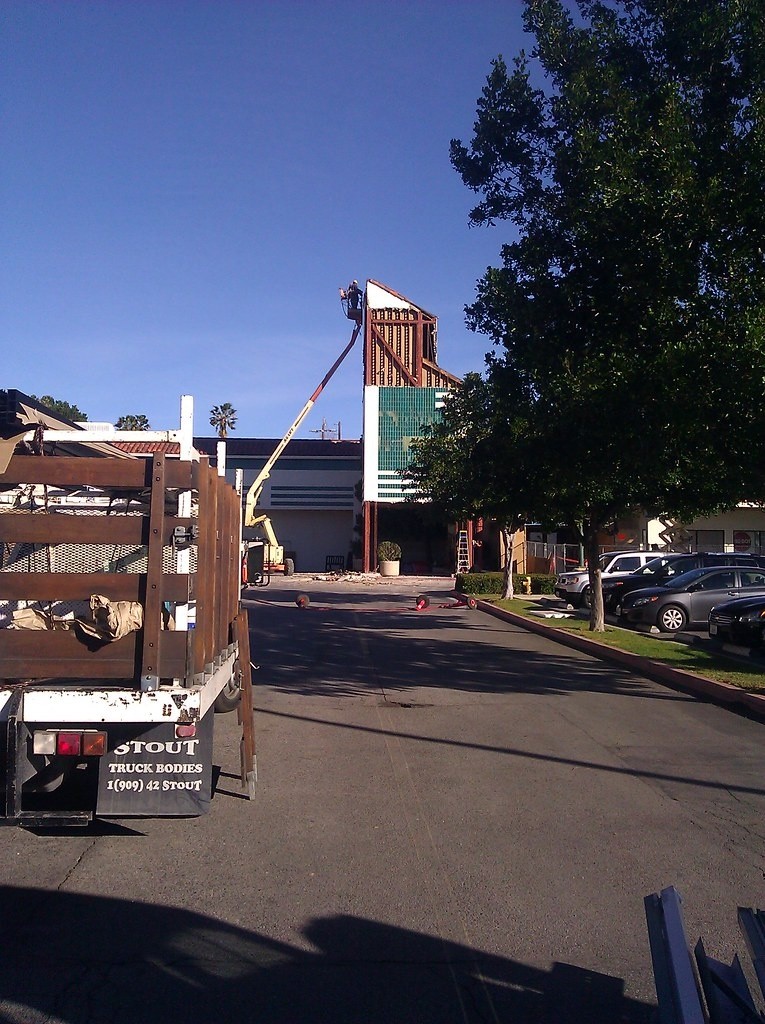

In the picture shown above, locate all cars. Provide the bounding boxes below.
[706,595,765,655]
[614,566,765,634]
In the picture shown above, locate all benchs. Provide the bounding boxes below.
[324,556,345,571]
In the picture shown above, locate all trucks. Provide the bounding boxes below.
[2,394,274,825]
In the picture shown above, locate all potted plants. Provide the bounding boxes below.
[376,541,402,576]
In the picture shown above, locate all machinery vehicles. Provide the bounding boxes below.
[239,292,365,576]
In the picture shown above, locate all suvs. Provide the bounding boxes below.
[554,553,765,612]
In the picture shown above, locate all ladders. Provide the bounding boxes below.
[454,531,472,575]
